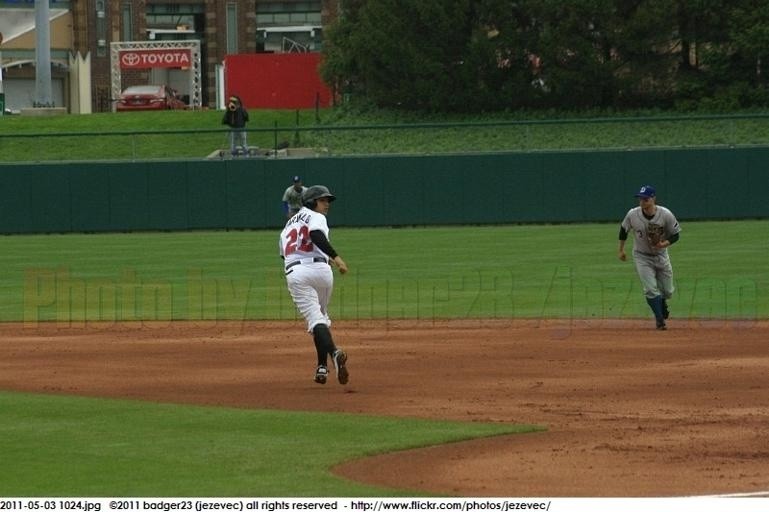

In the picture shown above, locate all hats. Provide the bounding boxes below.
[116,83,187,111]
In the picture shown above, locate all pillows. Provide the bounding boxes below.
[293,175,300,182]
[302,185,336,202]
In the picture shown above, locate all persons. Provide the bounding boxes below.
[617,183,682,330]
[282,175,308,219]
[279,184,350,385]
[222,96,249,156]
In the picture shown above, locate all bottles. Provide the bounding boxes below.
[631,186,657,199]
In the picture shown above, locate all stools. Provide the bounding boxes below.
[286,257,326,270]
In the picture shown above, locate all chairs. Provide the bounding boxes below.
[334,351,349,385]
[315,367,329,384]
